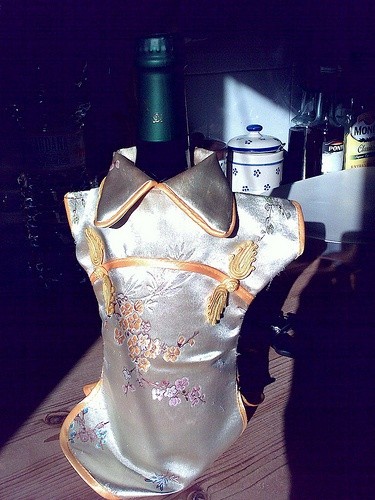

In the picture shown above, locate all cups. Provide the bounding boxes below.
[226,124,286,198]
[193,133,228,179]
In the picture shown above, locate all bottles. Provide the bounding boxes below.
[131,32,191,184]
[335,57,375,171]
[283,64,344,184]
